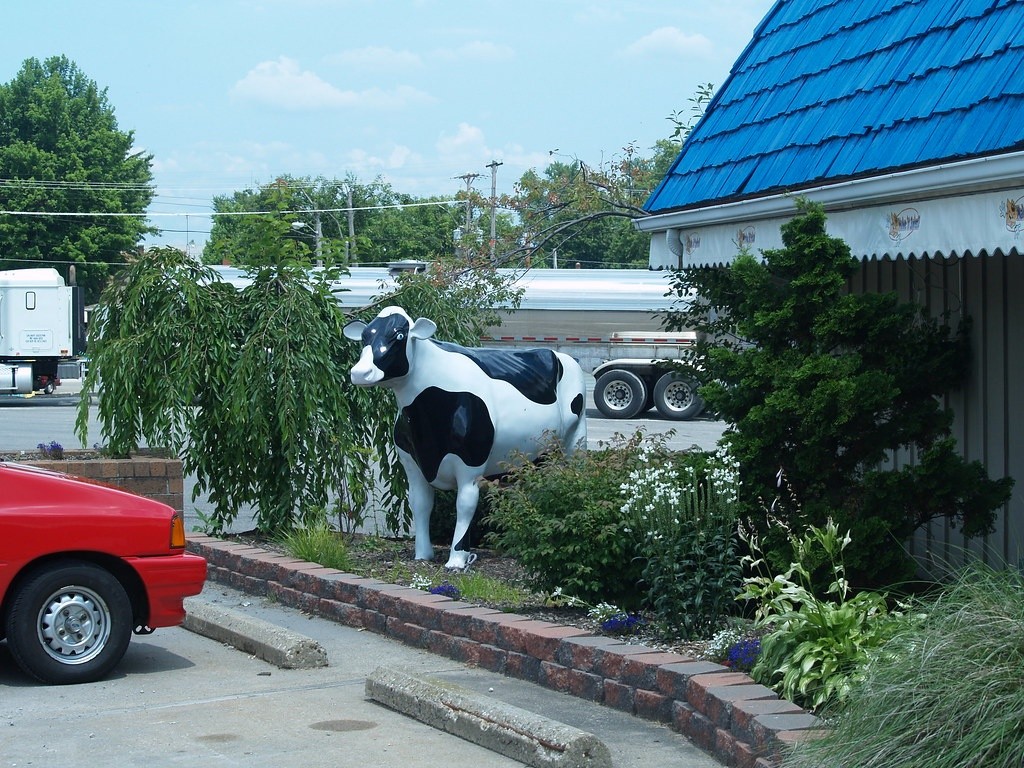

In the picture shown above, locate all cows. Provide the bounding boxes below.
[342,305,588,572]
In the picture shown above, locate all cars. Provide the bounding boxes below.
[0,461,208,686]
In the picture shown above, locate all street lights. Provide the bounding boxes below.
[291,221,323,267]
